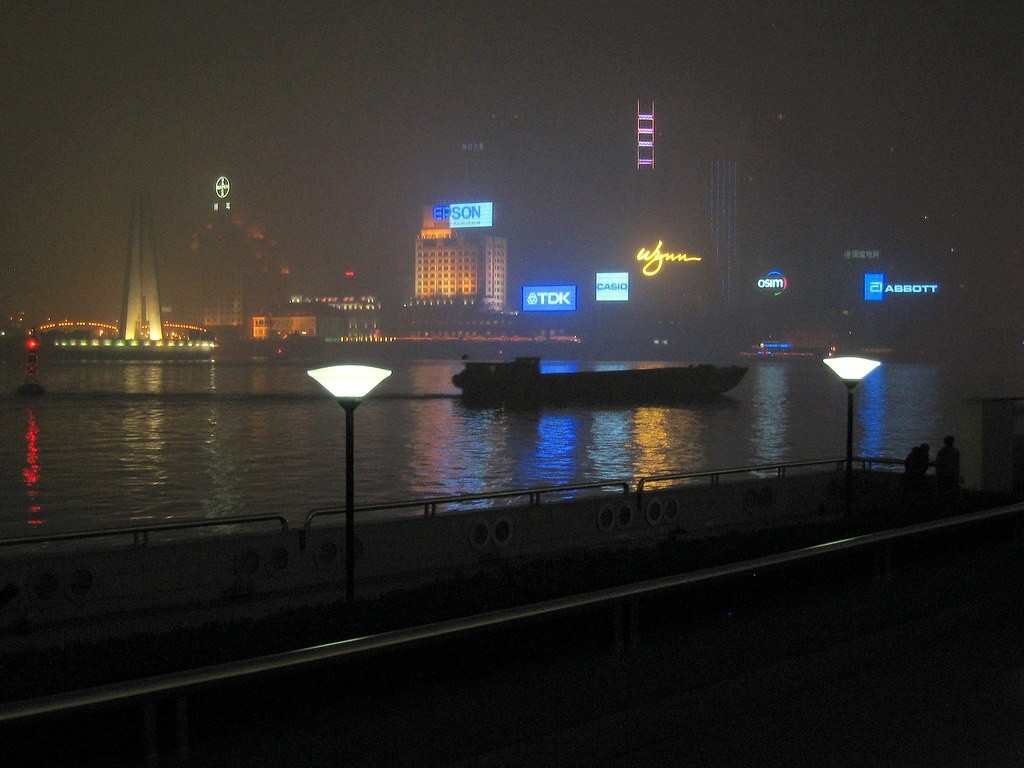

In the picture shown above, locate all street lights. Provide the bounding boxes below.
[822,355,882,522]
[305,363,393,603]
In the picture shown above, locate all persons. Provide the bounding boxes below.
[902,443,930,501]
[936,436,960,503]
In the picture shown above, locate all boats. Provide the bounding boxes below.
[16,327,47,395]
[448,354,752,402]
